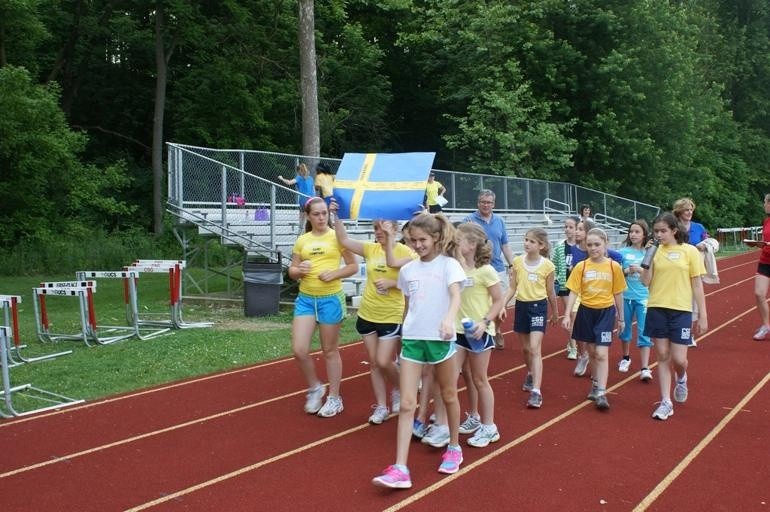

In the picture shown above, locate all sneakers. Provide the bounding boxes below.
[412,413,451,447]
[494,330,505,351]
[753,326,769,340]
[466,424,501,447]
[317,396,345,418]
[566,341,577,361]
[673,371,689,403]
[618,358,631,373]
[587,378,610,410]
[521,371,542,408]
[391,388,402,413]
[368,405,391,425]
[573,352,590,377]
[651,399,675,421]
[459,413,482,434]
[639,368,653,382]
[370,465,413,489]
[304,383,328,414]
[437,444,463,474]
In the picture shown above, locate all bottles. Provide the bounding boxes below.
[460,318,484,354]
[360,262,366,278]
[641,240,659,269]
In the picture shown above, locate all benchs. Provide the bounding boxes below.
[181,200,634,306]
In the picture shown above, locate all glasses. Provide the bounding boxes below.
[478,202,494,205]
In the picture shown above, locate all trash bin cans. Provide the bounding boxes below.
[242,249,284,317]
